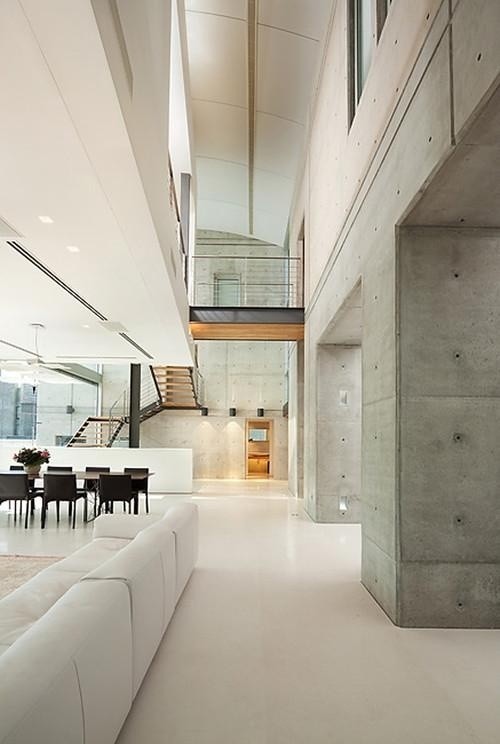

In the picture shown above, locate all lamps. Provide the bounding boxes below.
[230,408,235,417]
[201,407,207,416]
[257,408,263,417]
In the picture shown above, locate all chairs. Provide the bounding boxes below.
[0,465,156,529]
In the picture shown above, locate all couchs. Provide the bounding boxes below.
[0,499,200,744]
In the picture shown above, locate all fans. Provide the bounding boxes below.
[0,324,71,379]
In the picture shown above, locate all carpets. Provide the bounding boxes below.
[0,554,63,602]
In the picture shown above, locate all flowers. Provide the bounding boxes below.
[13,446,51,466]
